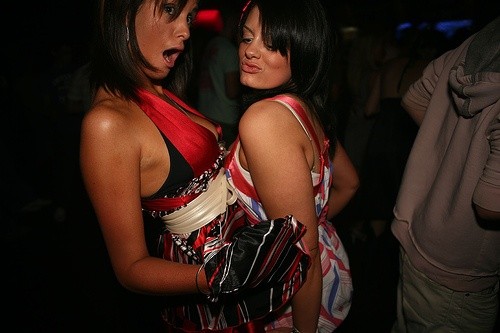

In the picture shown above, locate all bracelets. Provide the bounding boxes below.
[195,263,211,295]
[291,326,300,333]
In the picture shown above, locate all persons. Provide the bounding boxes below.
[0,3,452,258]
[223,0,360,333]
[79,0,311,333]
[390,16,500,333]
